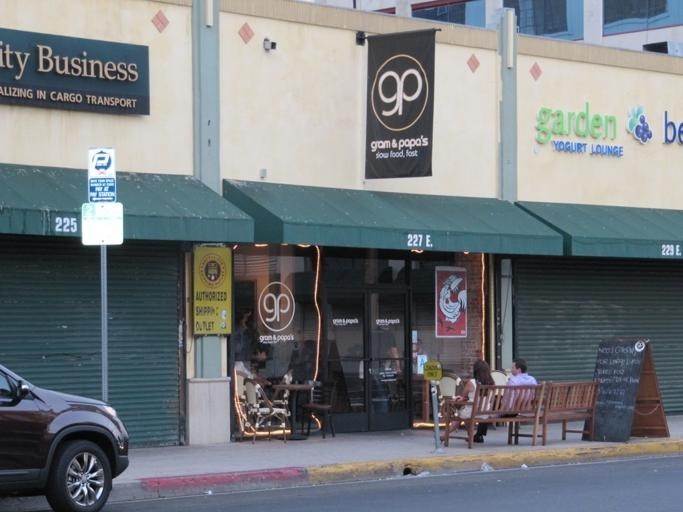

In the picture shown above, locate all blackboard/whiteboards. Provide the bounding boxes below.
[581,337,648,442]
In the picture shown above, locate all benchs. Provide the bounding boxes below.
[515,376,601,446]
[443,381,545,449]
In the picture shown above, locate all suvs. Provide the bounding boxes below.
[0,364,132,511]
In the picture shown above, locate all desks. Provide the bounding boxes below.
[273,383,312,440]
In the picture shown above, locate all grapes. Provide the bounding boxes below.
[636,115,652,142]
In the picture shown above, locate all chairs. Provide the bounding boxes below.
[302,379,342,439]
[241,378,289,444]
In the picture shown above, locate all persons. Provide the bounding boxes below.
[255,344,285,378]
[272,350,315,411]
[440,359,493,441]
[473,359,537,444]
[384,344,405,371]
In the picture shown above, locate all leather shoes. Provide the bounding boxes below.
[465,434,485,443]
